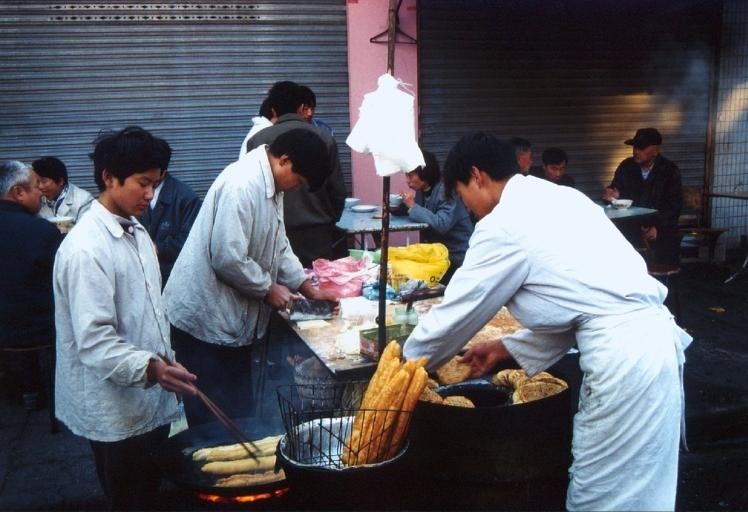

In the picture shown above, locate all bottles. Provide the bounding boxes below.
[392,305,418,326]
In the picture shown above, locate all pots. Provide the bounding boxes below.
[415,376,573,418]
[151,415,289,498]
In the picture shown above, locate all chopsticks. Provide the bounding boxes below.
[154,350,260,465]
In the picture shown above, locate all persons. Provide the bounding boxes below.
[296,85,333,136]
[160,128,329,426]
[238,97,279,160]
[54,127,200,512]
[400,129,694,512]
[133,138,202,294]
[530,147,576,188]
[601,128,682,267]
[0,158,68,413]
[32,156,94,235]
[246,79,345,273]
[509,137,534,175]
[400,151,474,286]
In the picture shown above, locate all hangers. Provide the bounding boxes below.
[370,27,417,44]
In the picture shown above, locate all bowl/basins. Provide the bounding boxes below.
[345,197,360,207]
[610,199,633,211]
[47,216,74,227]
[389,194,403,208]
[278,415,412,486]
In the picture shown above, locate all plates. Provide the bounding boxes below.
[352,205,377,213]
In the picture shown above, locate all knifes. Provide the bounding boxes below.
[281,299,338,320]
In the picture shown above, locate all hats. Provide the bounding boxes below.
[624,128,662,148]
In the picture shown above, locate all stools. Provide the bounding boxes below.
[679,229,736,284]
[0,342,58,431]
[649,264,682,316]
